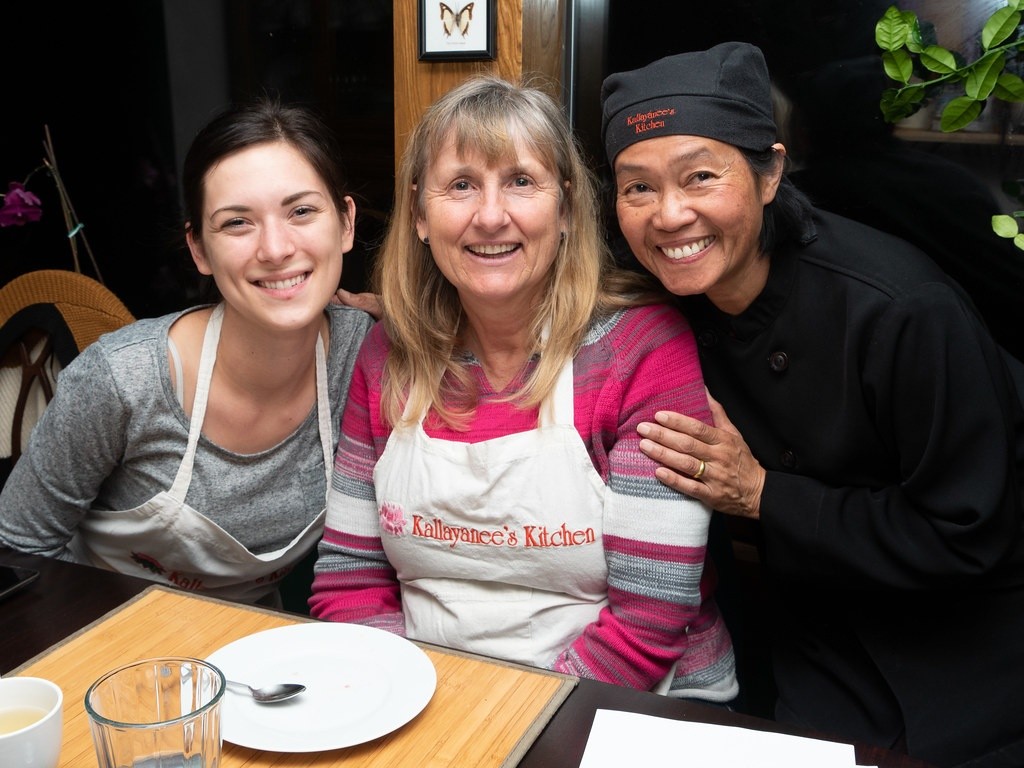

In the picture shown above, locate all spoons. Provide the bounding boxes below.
[224,680,306,704]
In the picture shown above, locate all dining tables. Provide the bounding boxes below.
[0,544,948,768]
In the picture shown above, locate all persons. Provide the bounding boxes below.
[309,41,1024,768]
[0,94,377,621]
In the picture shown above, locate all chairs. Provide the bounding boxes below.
[0,271,141,481]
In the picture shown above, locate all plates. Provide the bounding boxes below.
[202,622,437,753]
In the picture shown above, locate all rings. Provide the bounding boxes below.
[693,459,705,479]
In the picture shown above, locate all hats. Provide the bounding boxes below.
[599,42,778,174]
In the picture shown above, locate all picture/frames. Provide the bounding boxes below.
[415,0,498,62]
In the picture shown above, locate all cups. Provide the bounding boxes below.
[0,677,63,768]
[85,658,226,767]
